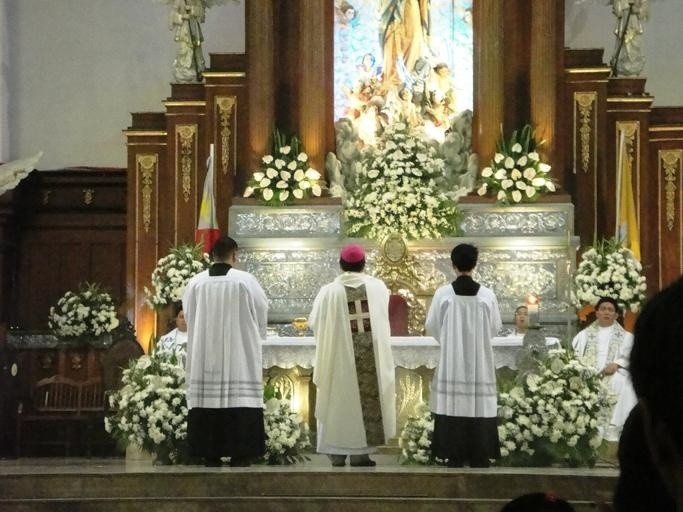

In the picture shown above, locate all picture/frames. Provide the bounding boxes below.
[324,0,479,194]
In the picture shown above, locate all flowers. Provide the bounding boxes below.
[477,121,562,207]
[337,113,472,241]
[398,340,617,469]
[104,329,186,467]
[574,226,647,317]
[142,233,214,312]
[243,128,329,208]
[263,384,310,464]
[47,281,137,346]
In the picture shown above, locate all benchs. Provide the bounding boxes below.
[13,376,123,457]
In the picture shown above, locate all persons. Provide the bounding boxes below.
[423,245,500,470]
[612,279,682,511]
[571,296,636,466]
[308,248,395,469]
[512,305,528,342]
[161,311,188,366]
[182,236,266,468]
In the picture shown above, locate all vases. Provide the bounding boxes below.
[268,453,278,464]
[534,438,553,466]
[152,445,172,465]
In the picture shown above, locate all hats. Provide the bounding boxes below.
[341,243,366,264]
